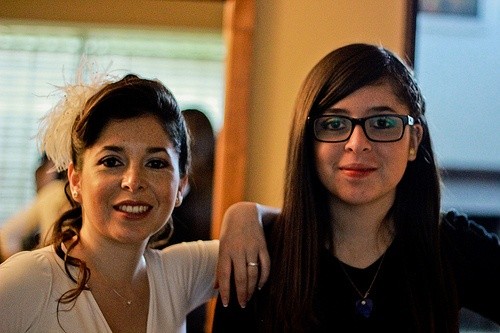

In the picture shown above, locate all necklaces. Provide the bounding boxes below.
[345,246,388,318]
[88,258,146,305]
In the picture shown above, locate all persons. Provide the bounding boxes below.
[211,43,500,333]
[0,73,283,333]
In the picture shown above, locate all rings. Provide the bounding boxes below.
[247,262,258,266]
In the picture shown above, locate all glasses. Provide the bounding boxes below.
[308,114,414,142]
